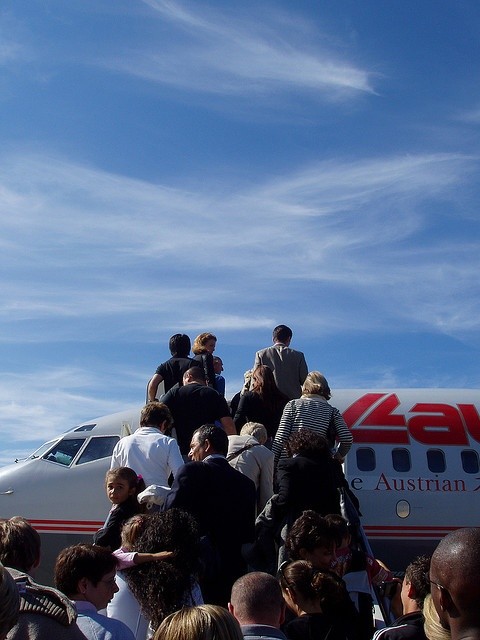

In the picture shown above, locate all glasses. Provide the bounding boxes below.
[277,560,296,599]
[426,576,445,588]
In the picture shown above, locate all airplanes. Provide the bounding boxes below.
[0,386,480,585]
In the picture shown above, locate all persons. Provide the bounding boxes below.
[92,466,146,548]
[267,428,346,530]
[281,558,353,639]
[430,526,480,640]
[152,603,244,640]
[322,513,353,574]
[146,333,201,402]
[227,572,288,640]
[192,332,217,390]
[212,355,226,398]
[230,391,239,418]
[271,370,353,472]
[111,545,175,570]
[227,421,275,513]
[53,541,137,640]
[372,555,430,640]
[108,401,185,488]
[0,516,86,640]
[423,592,452,640]
[163,423,257,600]
[281,508,338,572]
[106,513,206,640]
[232,364,291,436]
[249,324,309,398]
[160,367,236,463]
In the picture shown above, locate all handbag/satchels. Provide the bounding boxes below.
[327,407,339,446]
